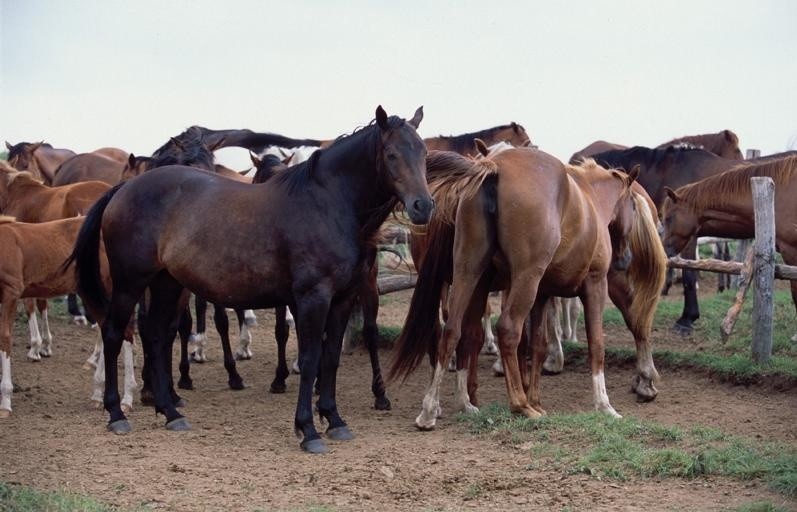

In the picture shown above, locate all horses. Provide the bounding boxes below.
[0,122,743,451]
[661,151,797,308]
